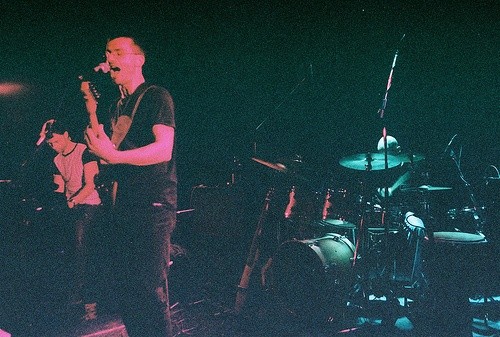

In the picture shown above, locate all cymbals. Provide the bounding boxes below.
[420,186,453,190]
[252,157,309,182]
[339,151,425,171]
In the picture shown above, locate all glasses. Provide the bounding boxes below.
[104,51,143,57]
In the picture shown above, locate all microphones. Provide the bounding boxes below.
[445,134,458,154]
[310,65,313,78]
[82,68,115,80]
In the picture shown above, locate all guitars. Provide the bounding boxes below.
[80,80,118,220]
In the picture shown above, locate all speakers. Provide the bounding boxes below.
[70,314,128,337]
[309,219,357,245]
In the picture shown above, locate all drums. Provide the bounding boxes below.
[268,232,362,316]
[447,207,488,233]
[422,232,487,281]
[312,219,358,245]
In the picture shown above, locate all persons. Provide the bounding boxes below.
[36,119,104,320]
[377,136,426,244]
[84,31,177,337]
